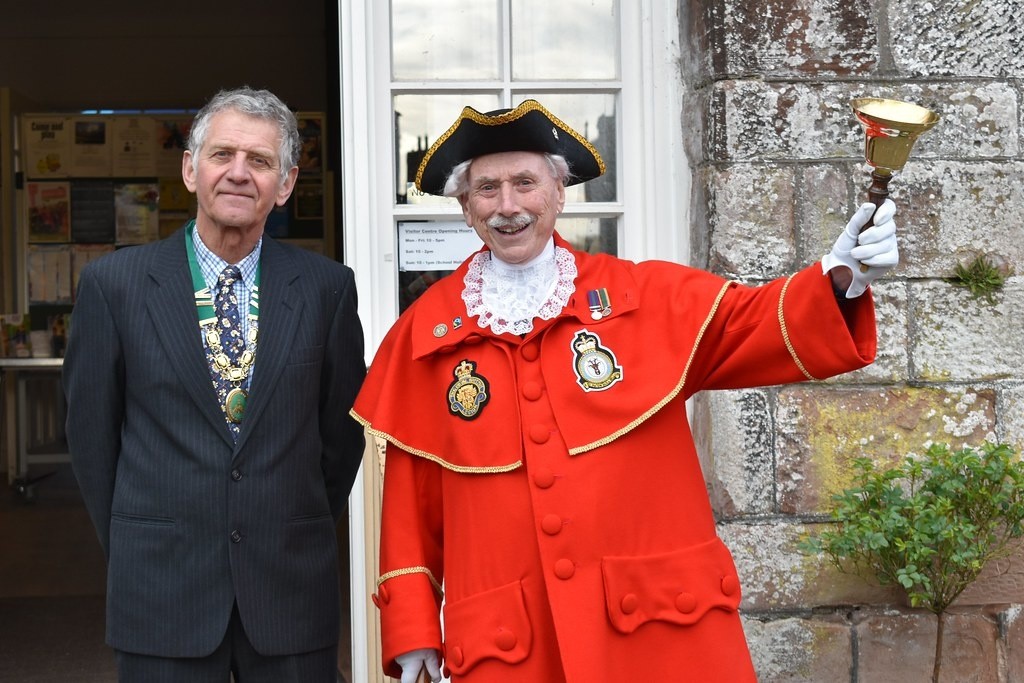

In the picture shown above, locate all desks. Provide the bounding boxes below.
[1,355,71,501]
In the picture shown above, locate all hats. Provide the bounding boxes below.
[414,99,606,197]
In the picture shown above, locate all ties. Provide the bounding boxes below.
[213,265,247,367]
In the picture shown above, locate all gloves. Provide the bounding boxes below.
[821,198,899,298]
[394,649,441,683]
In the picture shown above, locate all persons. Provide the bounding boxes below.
[350,96,901,682]
[57,84,370,683]
